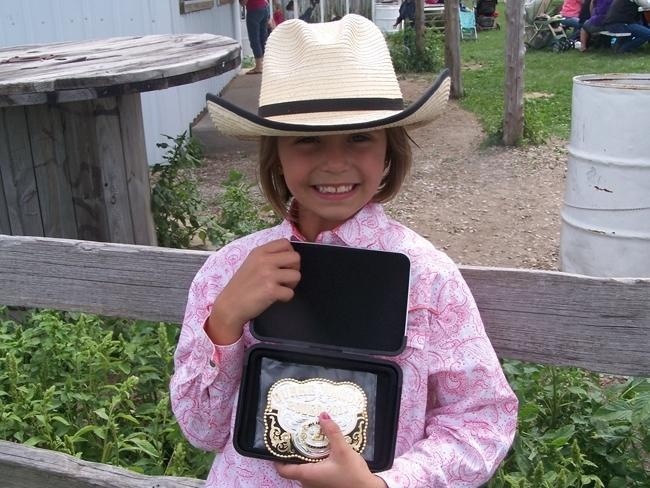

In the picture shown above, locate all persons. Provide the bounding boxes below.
[236,0,270,76]
[558,0,649,53]
[167,125,519,487]
[393,0,426,31]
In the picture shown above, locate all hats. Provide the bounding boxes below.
[206,13,453,136]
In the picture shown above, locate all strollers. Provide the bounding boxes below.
[474,0,501,33]
[524,0,576,55]
[458,1,479,43]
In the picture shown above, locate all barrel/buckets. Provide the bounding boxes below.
[373,4,400,33]
[559,72,650,278]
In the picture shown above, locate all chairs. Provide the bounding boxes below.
[459,6,479,42]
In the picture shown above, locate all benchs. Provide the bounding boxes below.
[588,31,635,52]
